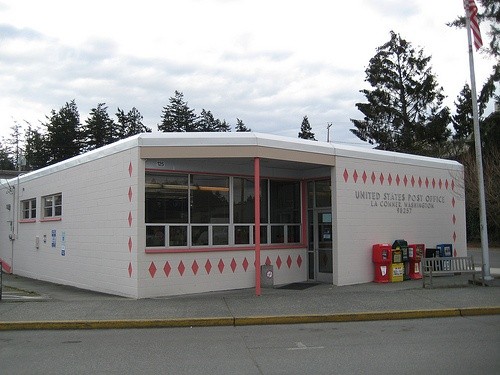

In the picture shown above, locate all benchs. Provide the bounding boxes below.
[421,255,487,289]
